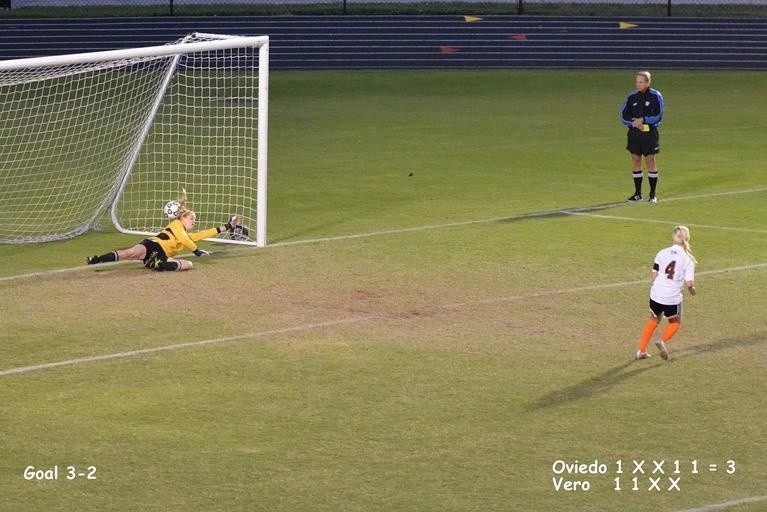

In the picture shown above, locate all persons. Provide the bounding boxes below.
[84,191,242,273]
[633,225,698,362]
[618,70,664,204]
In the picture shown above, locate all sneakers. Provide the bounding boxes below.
[654,339,668,361]
[86,256,95,265]
[144,251,158,270]
[648,196,658,203]
[628,194,643,202]
[636,350,652,360]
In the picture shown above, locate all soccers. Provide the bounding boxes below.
[164,200,183,220]
